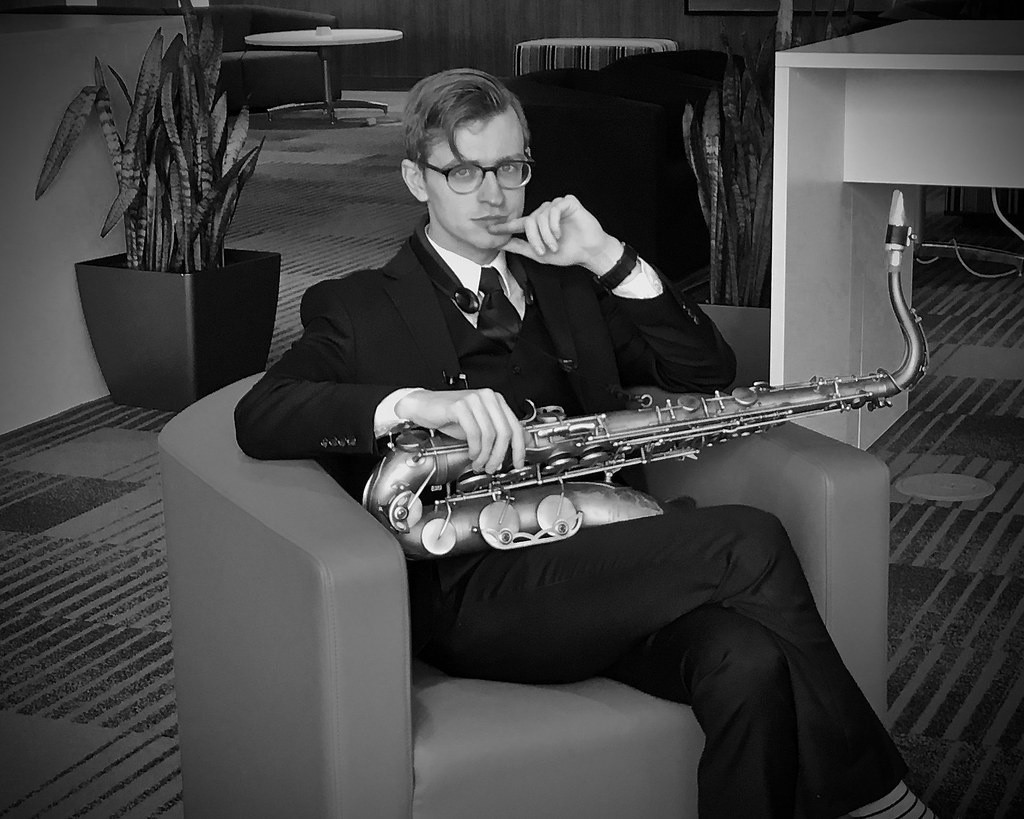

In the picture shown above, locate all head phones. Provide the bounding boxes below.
[408,231,535,314]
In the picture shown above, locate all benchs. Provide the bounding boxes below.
[513,37,679,77]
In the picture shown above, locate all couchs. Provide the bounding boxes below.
[503,50,746,278]
[154,370,892,819]
[212,4,342,116]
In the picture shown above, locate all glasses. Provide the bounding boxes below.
[410,149,535,194]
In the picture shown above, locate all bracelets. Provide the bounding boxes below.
[593,242,637,292]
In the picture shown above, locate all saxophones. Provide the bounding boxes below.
[362,190,929,558]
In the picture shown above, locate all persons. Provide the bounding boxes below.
[233,69,940,819]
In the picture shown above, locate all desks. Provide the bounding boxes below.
[764,20,1024,450]
[244,28,403,127]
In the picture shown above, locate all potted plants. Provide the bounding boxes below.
[33,0,283,412]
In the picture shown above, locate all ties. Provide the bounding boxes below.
[477,266,523,353]
